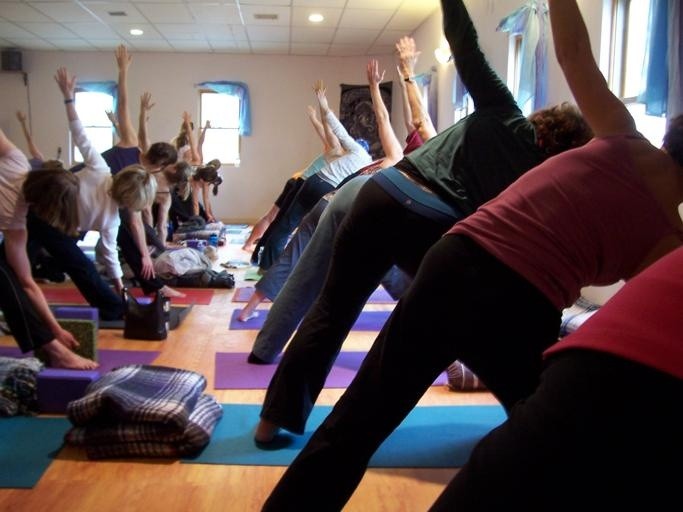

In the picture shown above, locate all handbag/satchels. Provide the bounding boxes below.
[121,285,169,341]
